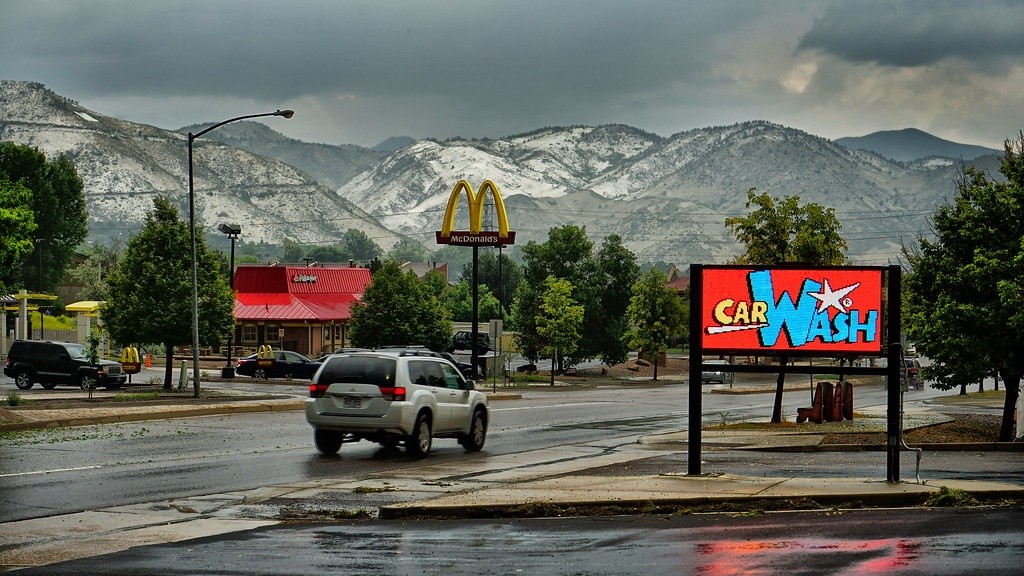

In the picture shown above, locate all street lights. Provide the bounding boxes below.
[303,257,312,268]
[217,223,242,378]
[187,108,295,398]
[494,243,508,355]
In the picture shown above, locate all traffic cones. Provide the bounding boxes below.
[145,353,151,367]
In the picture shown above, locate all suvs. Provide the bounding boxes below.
[452,331,490,355]
[4,338,128,392]
[901,359,924,391]
[306,345,491,463]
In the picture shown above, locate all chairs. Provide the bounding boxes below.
[796,381,853,424]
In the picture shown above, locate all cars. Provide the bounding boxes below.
[906,343,919,358]
[236,350,323,382]
[702,360,735,384]
